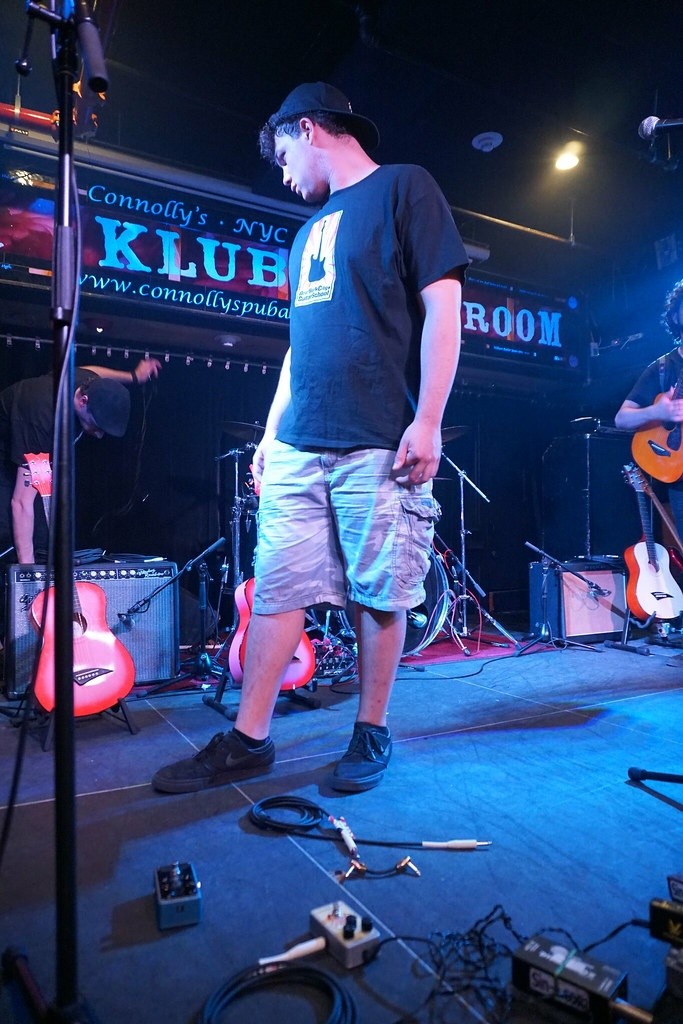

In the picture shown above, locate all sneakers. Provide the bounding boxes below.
[330,724,393,793]
[152,731,275,792]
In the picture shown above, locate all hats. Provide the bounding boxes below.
[88,378,131,437]
[276,82,380,154]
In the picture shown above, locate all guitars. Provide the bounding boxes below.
[631,370,683,484]
[23,454,137,715]
[621,464,683,621]
[228,464,315,690]
[636,467,683,570]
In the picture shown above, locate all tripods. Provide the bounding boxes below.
[126,536,228,699]
[515,541,604,656]
[437,444,523,652]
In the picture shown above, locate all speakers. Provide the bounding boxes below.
[178,585,222,646]
[4,561,180,699]
[529,558,634,646]
[541,432,666,560]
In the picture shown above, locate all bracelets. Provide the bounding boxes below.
[130,370,138,385]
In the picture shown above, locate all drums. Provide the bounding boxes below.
[340,547,449,657]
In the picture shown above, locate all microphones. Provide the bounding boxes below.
[638,116,683,140]
[410,612,428,628]
[589,590,611,596]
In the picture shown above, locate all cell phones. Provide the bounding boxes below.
[118,613,135,629]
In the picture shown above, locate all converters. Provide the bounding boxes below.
[513,940,630,1020]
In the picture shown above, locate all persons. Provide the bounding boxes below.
[614,280,683,544]
[0,357,162,565]
[149,83,474,795]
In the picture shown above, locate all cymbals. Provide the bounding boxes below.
[442,422,470,441]
[214,418,262,439]
